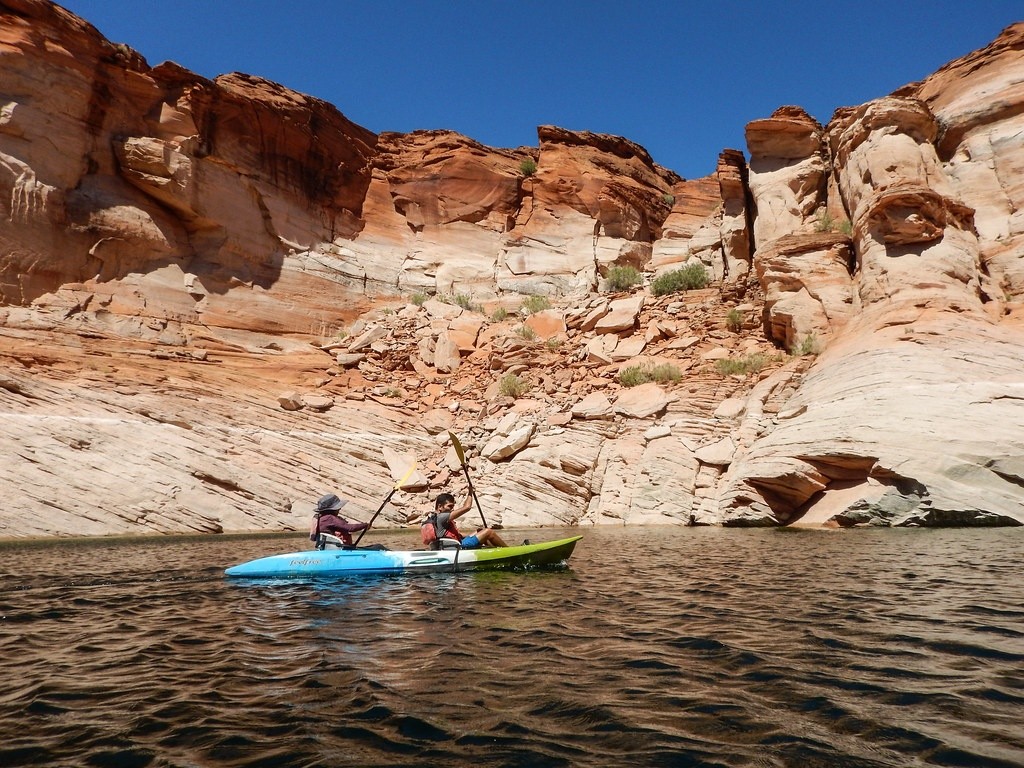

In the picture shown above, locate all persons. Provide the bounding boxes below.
[432,484,529,549]
[314,494,391,551]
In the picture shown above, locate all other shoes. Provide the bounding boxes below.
[521,539,530,546]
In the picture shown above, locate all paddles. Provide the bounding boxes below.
[447,430,486,529]
[354,460,418,546]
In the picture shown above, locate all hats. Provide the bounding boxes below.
[312,493,349,512]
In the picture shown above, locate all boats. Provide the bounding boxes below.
[223,533,585,581]
